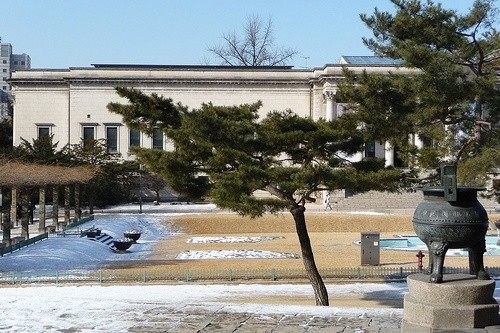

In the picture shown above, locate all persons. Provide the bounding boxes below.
[27,199,36,225]
[324,192,332,211]
[17,201,22,226]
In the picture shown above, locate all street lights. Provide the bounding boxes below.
[140,164,145,214]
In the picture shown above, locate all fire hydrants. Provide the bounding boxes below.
[416,250,425,273]
